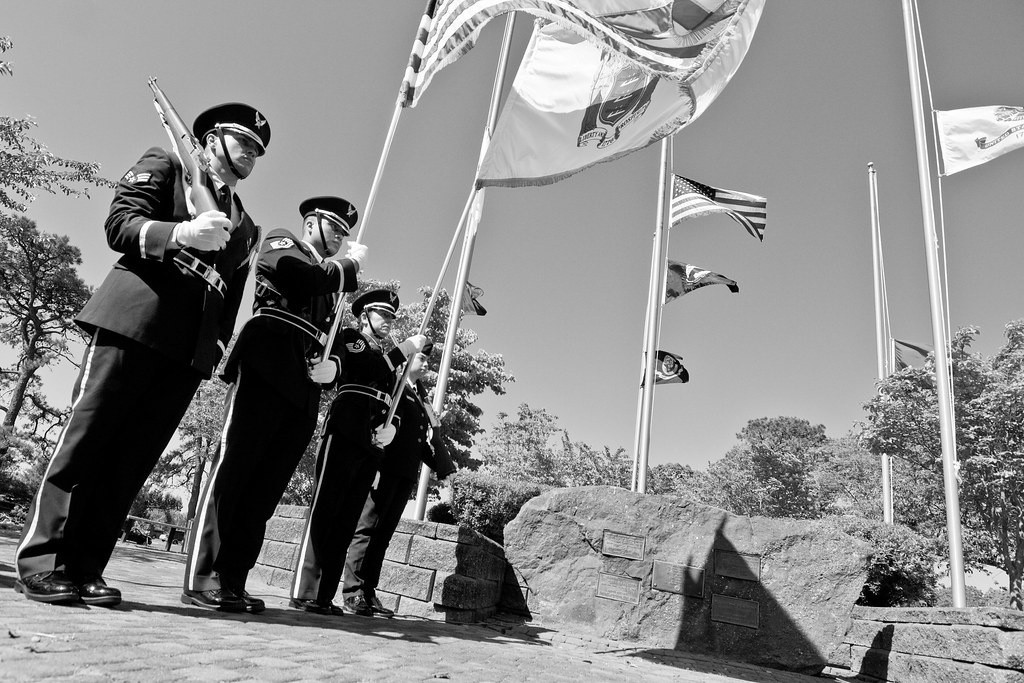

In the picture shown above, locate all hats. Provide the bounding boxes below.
[352,289,399,319]
[193,102,271,156]
[298,196,358,236]
[422,339,433,357]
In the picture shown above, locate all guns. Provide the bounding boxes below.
[146,74,225,230]
[414,380,457,484]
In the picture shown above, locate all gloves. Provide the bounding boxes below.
[345,240,369,272]
[375,422,396,446]
[176,209,232,251]
[310,356,337,383]
[399,335,427,357]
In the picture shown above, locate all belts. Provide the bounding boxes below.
[173,249,228,298]
[338,384,392,407]
[252,307,329,347]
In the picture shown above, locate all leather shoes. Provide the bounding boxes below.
[182,588,246,613]
[234,590,265,612]
[369,596,393,616]
[15,571,80,601]
[77,576,122,605]
[289,597,331,614]
[328,601,344,616]
[343,596,373,616]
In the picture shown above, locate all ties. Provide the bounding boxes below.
[221,184,232,220]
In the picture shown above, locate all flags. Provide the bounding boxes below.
[669,174,767,242]
[666,259,739,304]
[936,105,1024,175]
[641,350,689,387]
[473,1,769,189]
[398,0,750,107]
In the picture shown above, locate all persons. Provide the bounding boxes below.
[16,103,271,605]
[343,338,436,617]
[180,196,368,610]
[289,288,427,617]
